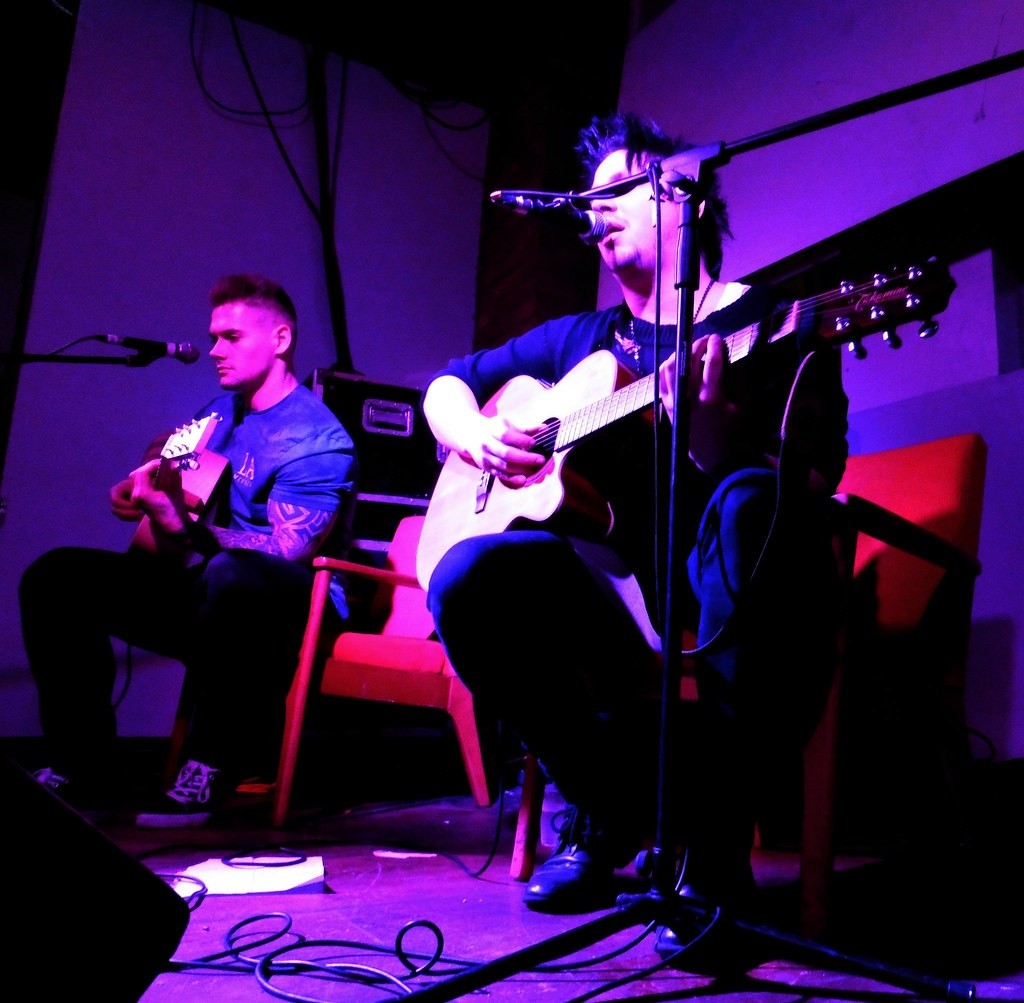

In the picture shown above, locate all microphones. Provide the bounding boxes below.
[97,334,200,364]
[491,191,608,246]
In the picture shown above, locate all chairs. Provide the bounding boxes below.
[508,432,989,944]
[154,511,491,830]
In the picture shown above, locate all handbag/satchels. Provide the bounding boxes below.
[848,726,1024,980]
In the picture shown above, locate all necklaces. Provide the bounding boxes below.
[628,276,719,376]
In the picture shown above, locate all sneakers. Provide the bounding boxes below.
[30,767,114,820]
[136,758,241,829]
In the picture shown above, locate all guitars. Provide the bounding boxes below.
[126,412,232,570]
[414,257,959,594]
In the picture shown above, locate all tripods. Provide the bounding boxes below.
[380,51,1024,1003]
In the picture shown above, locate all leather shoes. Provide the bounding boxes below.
[523,803,642,903]
[654,848,757,954]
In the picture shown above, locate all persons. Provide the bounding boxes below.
[13,263,354,833]
[422,107,854,968]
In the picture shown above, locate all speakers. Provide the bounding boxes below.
[0,756,191,1003]
